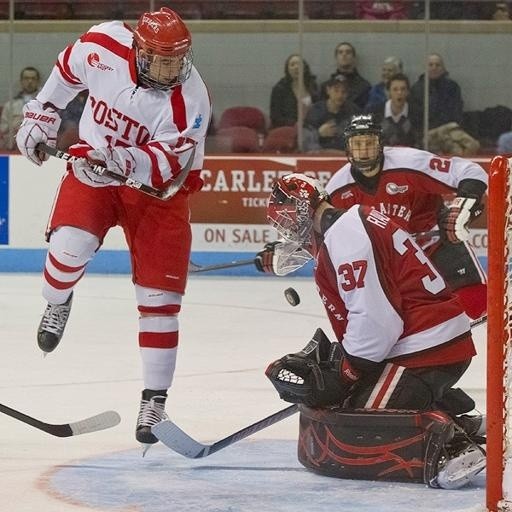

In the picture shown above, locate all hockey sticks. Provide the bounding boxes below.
[189,259,256,273]
[0,405,121,438]
[37,142,196,202]
[150,405,301,460]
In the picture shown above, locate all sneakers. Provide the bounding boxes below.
[38,289,74,352]
[437,420,476,472]
[136,389,168,443]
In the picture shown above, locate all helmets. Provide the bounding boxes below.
[342,112,385,172]
[133,7,193,96]
[263,171,331,261]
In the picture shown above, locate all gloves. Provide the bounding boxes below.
[254,240,280,277]
[264,327,364,410]
[68,143,137,188]
[13,100,62,168]
[434,187,480,249]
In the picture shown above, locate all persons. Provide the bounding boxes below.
[262,166,492,492]
[262,40,463,151]
[1,65,42,155]
[17,5,214,448]
[323,113,491,333]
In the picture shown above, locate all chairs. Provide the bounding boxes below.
[218,105,265,134]
[1,1,410,24]
[261,126,296,152]
[213,125,259,153]
